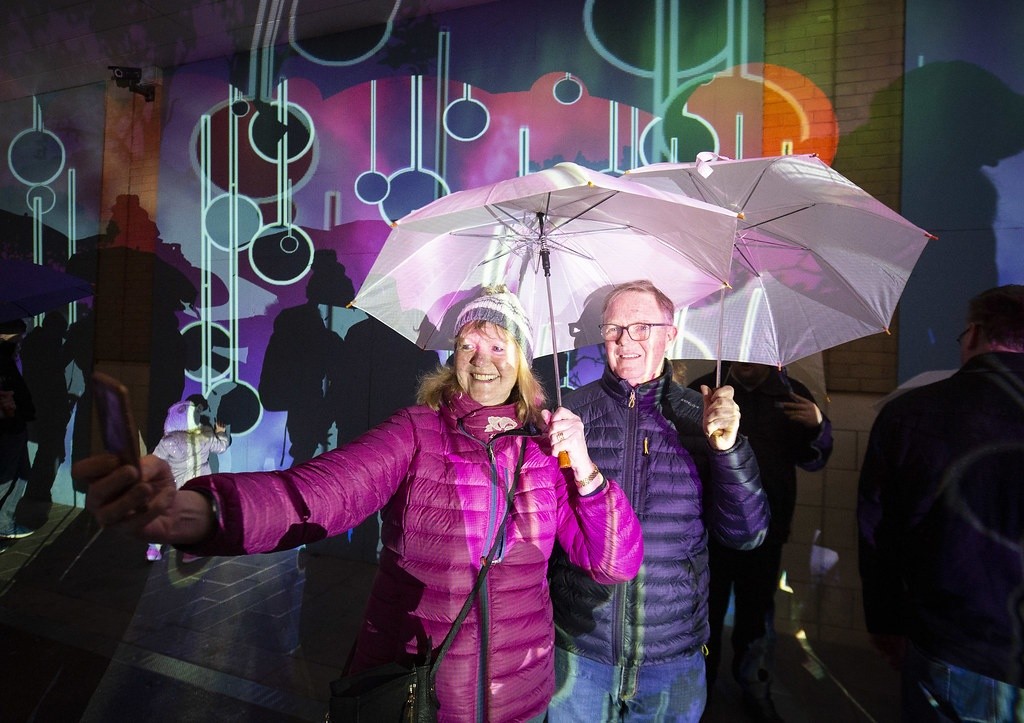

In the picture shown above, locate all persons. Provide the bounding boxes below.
[568,284,1024,723]
[532,282,771,723]
[0,319,36,554]
[147,401,230,564]
[70,282,644,723]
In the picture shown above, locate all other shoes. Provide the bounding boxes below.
[744,686,776,722]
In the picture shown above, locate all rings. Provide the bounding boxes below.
[556,430,564,442]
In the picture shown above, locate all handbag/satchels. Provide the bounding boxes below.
[325,656,440,723]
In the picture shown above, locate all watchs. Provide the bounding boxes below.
[210,492,220,528]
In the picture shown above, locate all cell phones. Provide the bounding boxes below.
[91,371,143,470]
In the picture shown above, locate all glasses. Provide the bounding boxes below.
[956,322,983,346]
[599,323,672,342]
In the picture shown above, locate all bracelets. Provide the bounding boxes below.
[573,463,600,488]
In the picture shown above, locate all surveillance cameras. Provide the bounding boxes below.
[107,65,142,83]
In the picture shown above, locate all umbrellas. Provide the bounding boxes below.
[0,254,96,324]
[676,351,831,420]
[617,151,938,441]
[345,162,746,470]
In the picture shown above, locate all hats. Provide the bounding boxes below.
[452,282,534,369]
